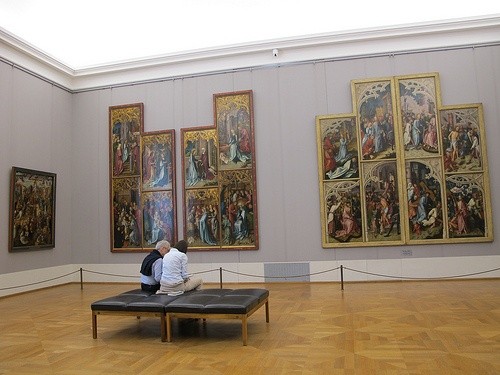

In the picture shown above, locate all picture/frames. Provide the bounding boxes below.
[9,167,56,252]
[395,72,494,245]
[316,77,406,248]
[180,90,259,252]
[109,103,179,253]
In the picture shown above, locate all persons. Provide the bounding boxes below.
[156,238,203,294]
[139,240,171,295]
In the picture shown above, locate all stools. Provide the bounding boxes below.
[91,289,197,341]
[165,289,269,346]
